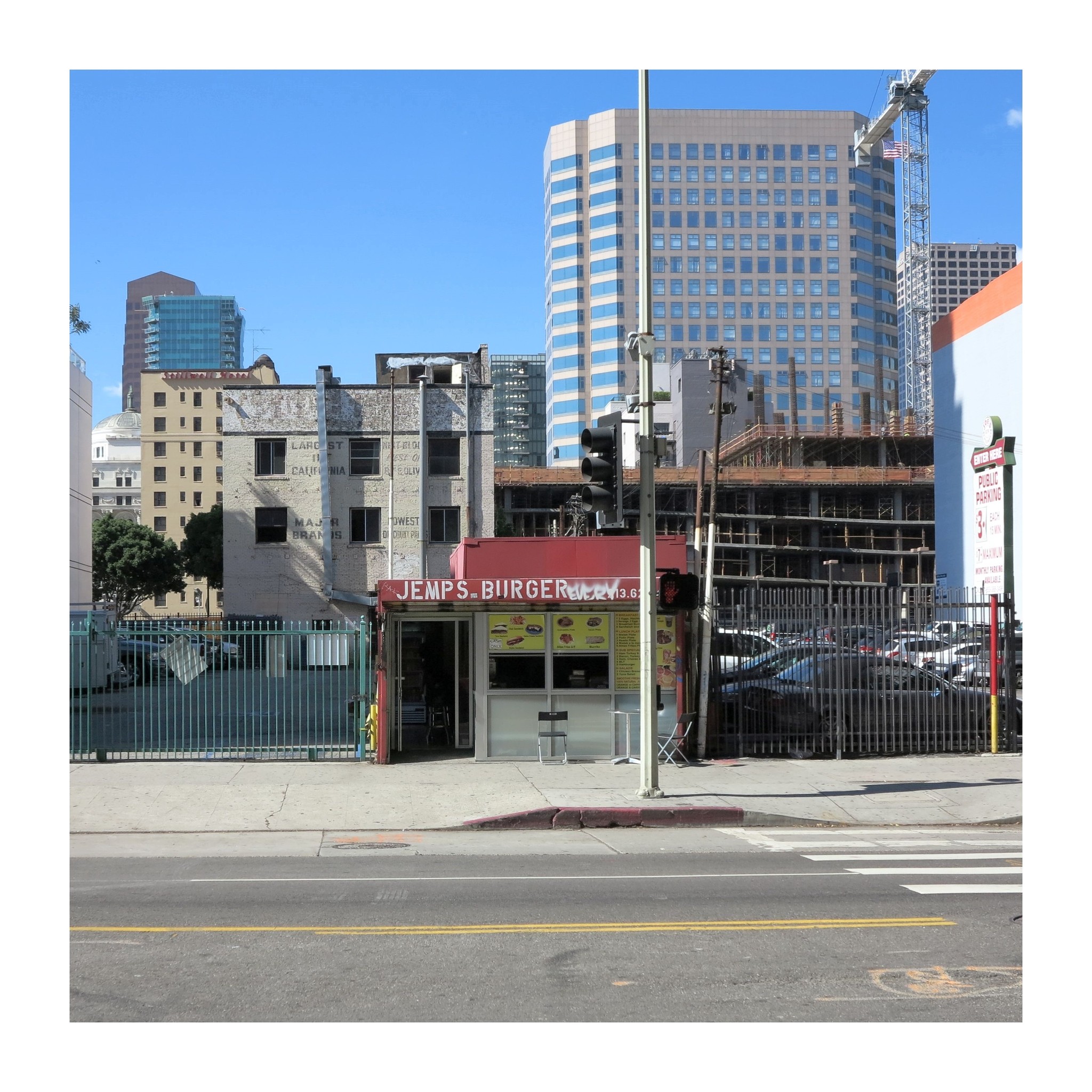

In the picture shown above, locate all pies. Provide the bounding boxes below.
[561,617,572,624]
[585,636,604,643]
[664,630,674,637]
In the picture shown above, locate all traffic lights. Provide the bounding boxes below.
[581,412,624,524]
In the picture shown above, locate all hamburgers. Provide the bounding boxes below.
[527,624,542,632]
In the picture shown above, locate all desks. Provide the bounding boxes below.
[606,708,641,764]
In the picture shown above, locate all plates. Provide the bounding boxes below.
[656,629,675,645]
[586,617,603,628]
[557,617,574,628]
[526,624,543,635]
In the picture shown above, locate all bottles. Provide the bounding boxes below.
[403,649,420,671]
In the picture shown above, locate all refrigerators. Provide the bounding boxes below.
[395,627,427,724]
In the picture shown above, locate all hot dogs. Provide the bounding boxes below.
[507,636,524,646]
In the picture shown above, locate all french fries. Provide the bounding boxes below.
[510,615,526,625]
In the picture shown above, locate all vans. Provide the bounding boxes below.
[974,633,1022,689]
[922,621,990,638]
[719,628,780,669]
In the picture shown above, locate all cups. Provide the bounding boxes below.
[665,616,673,627]
[656,666,676,689]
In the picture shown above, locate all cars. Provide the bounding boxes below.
[117,635,166,685]
[118,626,205,662]
[766,624,938,656]
[721,644,863,686]
[153,625,226,673]
[945,657,990,687]
[880,637,951,664]
[945,627,1005,645]
[182,628,242,670]
[913,643,981,681]
[721,653,1022,745]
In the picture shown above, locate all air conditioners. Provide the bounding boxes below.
[216,501,222,506]
[217,402,222,406]
[216,427,222,431]
[216,451,222,456]
[217,601,223,606]
[216,476,222,480]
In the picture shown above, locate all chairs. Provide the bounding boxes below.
[537,711,568,765]
[658,711,696,768]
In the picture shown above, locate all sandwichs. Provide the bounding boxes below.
[490,623,508,634]
